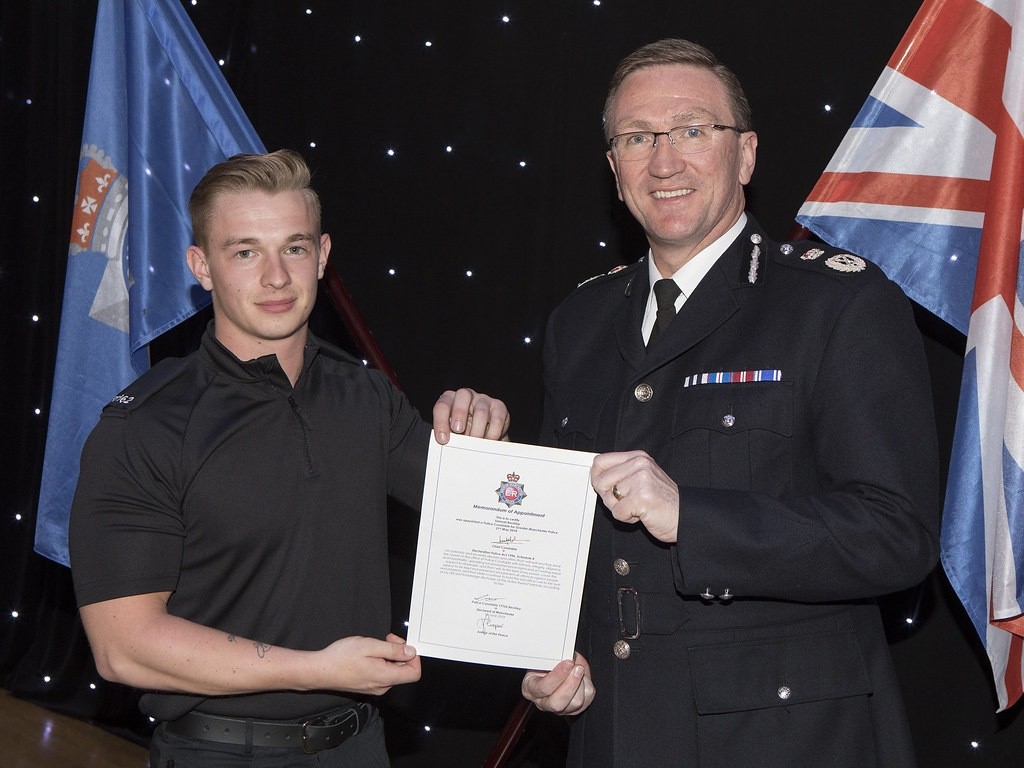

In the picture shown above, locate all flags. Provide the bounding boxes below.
[33,0,266,568]
[792,1,1024,713]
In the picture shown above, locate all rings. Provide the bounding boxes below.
[612,484,624,500]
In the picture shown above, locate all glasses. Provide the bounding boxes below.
[609,124,747,159]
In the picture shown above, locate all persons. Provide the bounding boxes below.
[522,36,947,768]
[68,150,510,768]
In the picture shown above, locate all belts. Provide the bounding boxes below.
[165,705,374,753]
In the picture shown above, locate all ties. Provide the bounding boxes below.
[646,278,682,347]
